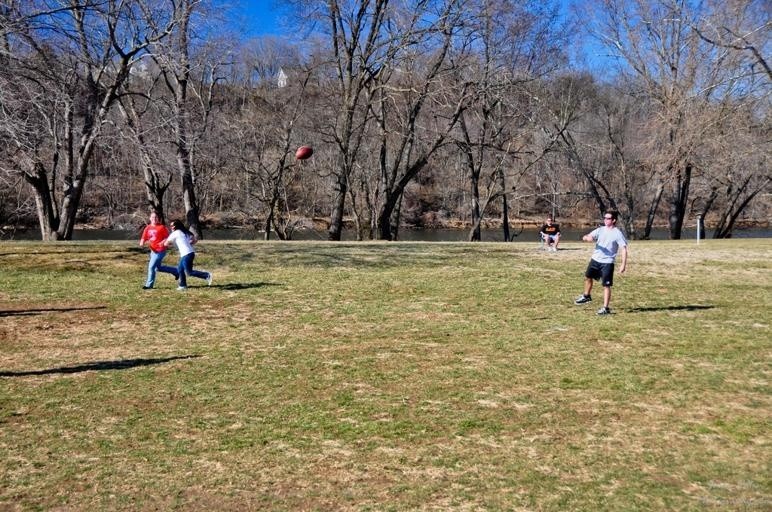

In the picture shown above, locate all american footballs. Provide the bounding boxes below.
[294,145,313,159]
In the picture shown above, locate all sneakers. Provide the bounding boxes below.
[175,273,179,280]
[576,295,591,303]
[176,285,187,290]
[596,307,610,315]
[143,286,153,289]
[548,246,557,252]
[206,272,213,285]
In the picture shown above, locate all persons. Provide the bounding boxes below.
[164,219,213,290]
[538,218,561,252]
[573,212,628,315]
[139,211,179,289]
